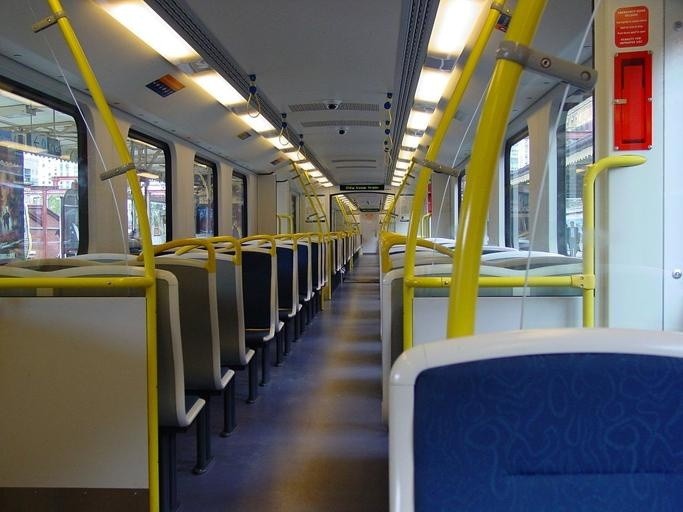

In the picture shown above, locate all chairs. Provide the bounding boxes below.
[379,232,583,340]
[387,328,683,511]
[0,231,364,471]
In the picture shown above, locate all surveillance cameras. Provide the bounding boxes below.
[338,127,348,135]
[324,100,341,110]
